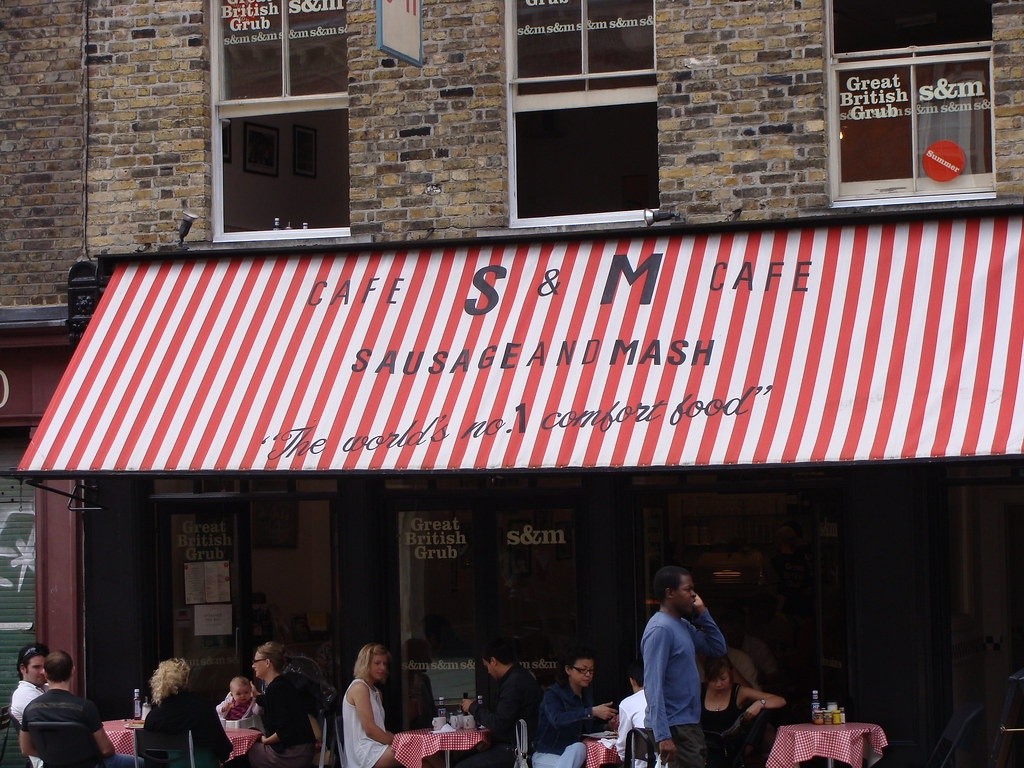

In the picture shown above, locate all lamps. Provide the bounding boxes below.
[644,207,686,226]
[177,211,199,250]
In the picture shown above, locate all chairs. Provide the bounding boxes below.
[925,704,984,768]
[0,703,771,768]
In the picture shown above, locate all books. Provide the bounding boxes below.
[702,713,744,739]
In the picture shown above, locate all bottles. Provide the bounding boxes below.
[811,690,845,725]
[133,688,142,720]
[437,696,447,718]
[141,703,151,720]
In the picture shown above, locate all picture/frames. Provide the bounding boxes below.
[222,118,232,165]
[243,121,280,178]
[292,124,317,180]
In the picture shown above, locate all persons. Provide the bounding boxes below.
[10,643,49,768]
[424,614,496,705]
[454,637,539,768]
[342,642,445,768]
[248,640,316,768]
[394,638,439,728]
[639,565,728,768]
[615,660,668,768]
[18,651,144,768]
[215,677,260,720]
[701,655,786,768]
[532,650,619,768]
[144,657,233,768]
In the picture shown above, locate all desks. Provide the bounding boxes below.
[581,737,626,768]
[766,722,889,768]
[390,727,494,768]
[100,719,264,768]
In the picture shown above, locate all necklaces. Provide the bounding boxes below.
[705,692,729,711]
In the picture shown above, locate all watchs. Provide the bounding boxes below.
[760,698,766,708]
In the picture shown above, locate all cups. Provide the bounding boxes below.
[450,716,461,730]
[457,714,476,729]
[432,716,447,731]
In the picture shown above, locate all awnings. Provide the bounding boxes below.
[16,214,1024,474]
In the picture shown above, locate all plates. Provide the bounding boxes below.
[582,732,618,739]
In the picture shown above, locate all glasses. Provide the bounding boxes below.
[23,647,40,657]
[569,663,599,676]
[250,655,268,664]
